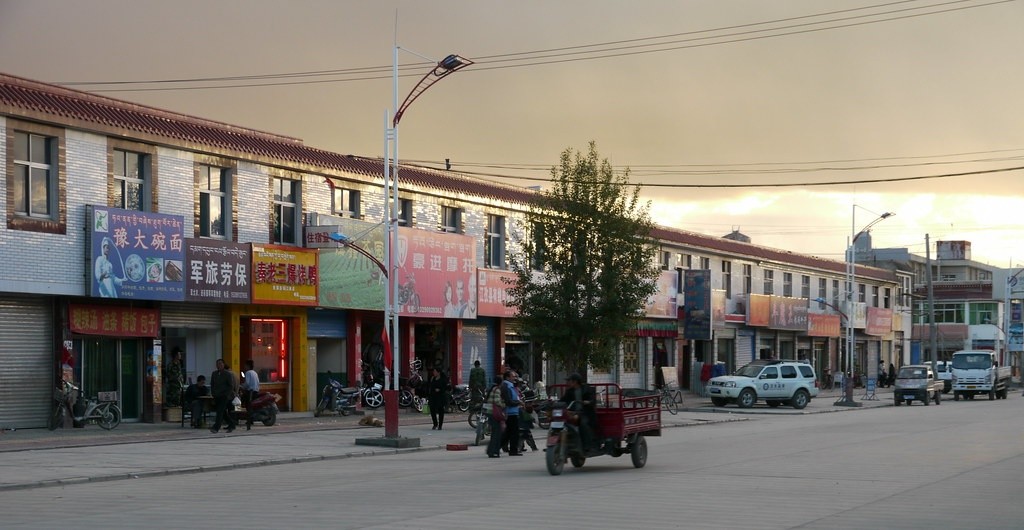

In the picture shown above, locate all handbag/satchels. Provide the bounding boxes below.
[231,396,241,406]
[492,388,507,420]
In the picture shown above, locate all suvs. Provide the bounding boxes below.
[705,359,819,411]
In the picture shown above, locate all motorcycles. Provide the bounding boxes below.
[313,370,365,416]
[541,382,663,477]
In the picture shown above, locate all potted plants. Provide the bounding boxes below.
[163,352,185,423]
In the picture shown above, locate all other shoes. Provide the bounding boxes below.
[211,429,217,433]
[432,424,438,430]
[521,448,527,451]
[532,448,538,451]
[510,453,522,456]
[488,453,499,458]
[227,428,233,432]
[438,426,442,430]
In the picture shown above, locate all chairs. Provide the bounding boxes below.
[229,389,254,429]
[175,377,207,428]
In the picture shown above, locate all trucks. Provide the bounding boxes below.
[893,365,945,406]
[949,350,1012,401]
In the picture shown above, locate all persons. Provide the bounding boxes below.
[799,354,810,364]
[166,346,183,406]
[239,359,260,424]
[185,375,209,429]
[146,368,152,382]
[558,373,596,450]
[469,360,486,414]
[425,367,447,430]
[486,368,539,458]
[94,237,122,298]
[444,274,477,319]
[208,359,236,433]
[149,353,153,362]
[878,360,895,388]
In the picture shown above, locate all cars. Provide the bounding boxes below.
[922,360,954,394]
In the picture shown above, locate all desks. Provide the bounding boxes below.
[192,396,221,431]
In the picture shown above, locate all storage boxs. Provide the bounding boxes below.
[96,390,117,401]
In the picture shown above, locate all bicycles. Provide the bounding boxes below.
[595,388,605,407]
[640,379,678,415]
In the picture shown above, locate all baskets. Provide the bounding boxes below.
[55,388,72,403]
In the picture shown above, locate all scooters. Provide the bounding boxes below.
[229,371,280,427]
[47,376,122,431]
[364,372,549,430]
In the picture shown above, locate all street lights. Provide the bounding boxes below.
[812,204,897,408]
[377,40,477,441]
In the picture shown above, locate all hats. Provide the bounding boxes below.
[565,373,582,382]
